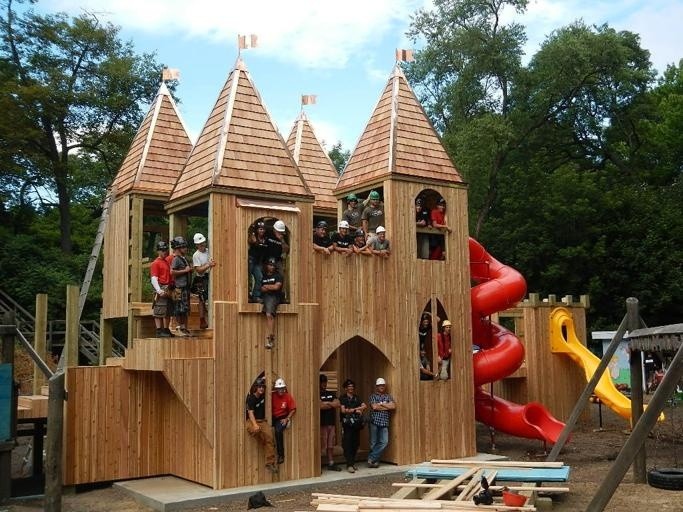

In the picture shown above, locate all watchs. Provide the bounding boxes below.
[286,418,289,422]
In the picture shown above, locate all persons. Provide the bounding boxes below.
[248,220,290,349]
[368,378,396,468]
[270,378,296,464]
[416,197,452,261]
[151,232,217,338]
[339,379,368,472]
[246,378,279,473]
[419,311,452,380]
[319,373,343,472]
[313,190,390,258]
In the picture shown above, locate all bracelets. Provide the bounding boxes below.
[353,409,356,414]
[380,401,383,406]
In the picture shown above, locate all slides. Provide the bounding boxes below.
[470,238,573,447]
[551,308,664,431]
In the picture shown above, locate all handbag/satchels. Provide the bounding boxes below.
[341,410,366,432]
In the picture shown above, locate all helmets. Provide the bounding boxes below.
[341,379,353,387]
[254,219,288,233]
[501,490,527,507]
[353,228,364,237]
[154,240,168,252]
[252,377,287,389]
[415,196,446,207]
[315,220,328,229]
[374,377,386,387]
[421,312,452,327]
[337,220,349,229]
[374,225,386,234]
[346,194,358,201]
[319,374,328,383]
[170,236,188,249]
[266,257,278,266]
[192,232,206,244]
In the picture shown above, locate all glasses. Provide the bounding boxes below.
[368,191,380,201]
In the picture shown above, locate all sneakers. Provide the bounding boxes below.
[327,463,341,471]
[163,327,175,337]
[174,325,187,337]
[199,319,208,329]
[251,294,264,304]
[276,455,284,464]
[180,327,195,337]
[372,459,380,468]
[366,457,374,468]
[346,465,355,473]
[155,327,170,337]
[264,461,279,473]
[264,333,275,349]
[247,295,253,303]
[352,463,359,470]
